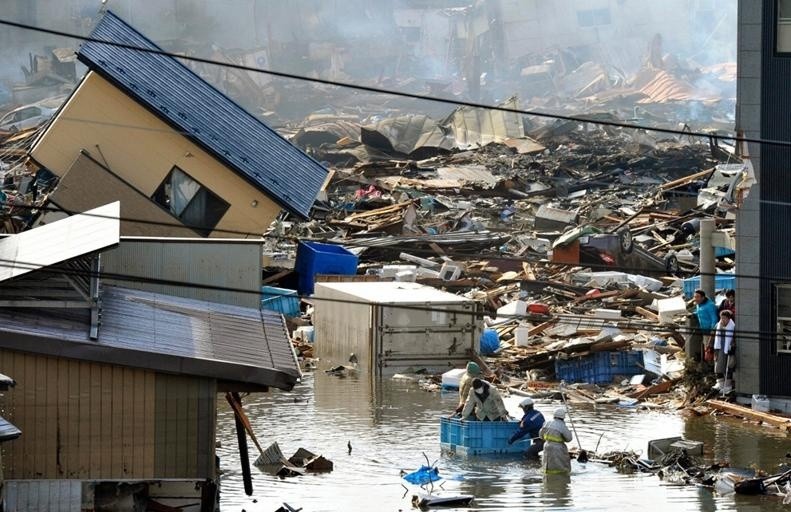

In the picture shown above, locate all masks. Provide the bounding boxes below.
[475,387,483,394]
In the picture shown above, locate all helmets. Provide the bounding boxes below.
[518,398,533,406]
[553,409,565,419]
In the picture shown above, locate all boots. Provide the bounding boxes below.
[712,379,732,394]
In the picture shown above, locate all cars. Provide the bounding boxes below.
[0,103,59,133]
[579,228,680,279]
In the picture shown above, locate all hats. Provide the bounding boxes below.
[473,379,482,389]
[468,363,481,376]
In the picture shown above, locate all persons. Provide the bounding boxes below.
[538,409,572,475]
[704,309,736,396]
[685,289,719,372]
[717,290,735,322]
[507,399,545,460]
[447,360,517,422]
